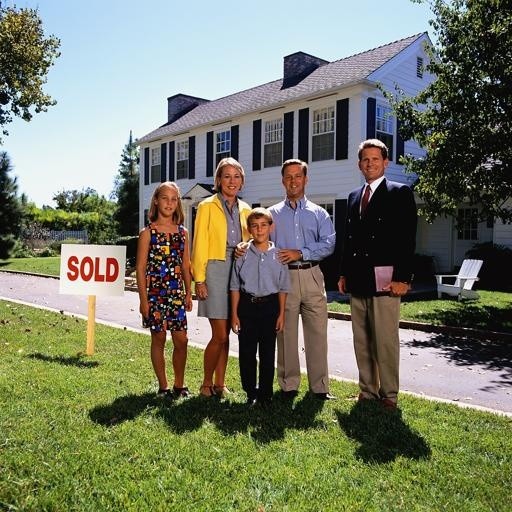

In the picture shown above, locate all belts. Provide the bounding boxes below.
[239,293,278,303]
[288,262,320,269]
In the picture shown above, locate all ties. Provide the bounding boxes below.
[361,185,371,215]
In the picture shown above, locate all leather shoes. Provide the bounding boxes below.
[344,393,366,400]
[382,399,396,409]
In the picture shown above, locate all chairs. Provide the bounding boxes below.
[434,255,485,301]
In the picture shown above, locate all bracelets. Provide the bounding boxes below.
[298,249,303,258]
[195,282,207,286]
[185,288,190,292]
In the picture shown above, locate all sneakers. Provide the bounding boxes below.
[158,385,338,400]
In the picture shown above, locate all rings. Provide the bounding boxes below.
[288,255,292,259]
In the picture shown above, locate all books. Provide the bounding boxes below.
[374,266,412,293]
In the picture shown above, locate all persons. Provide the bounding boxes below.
[337,138,417,412]
[192,157,253,398]
[230,207,290,408]
[136,181,191,400]
[234,159,337,399]
